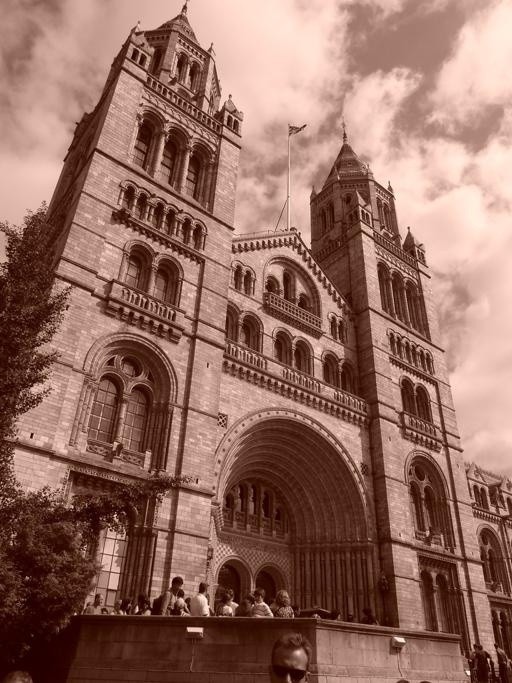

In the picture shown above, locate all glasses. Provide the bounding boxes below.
[272,664,306,681]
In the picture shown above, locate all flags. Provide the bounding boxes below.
[288,123,307,135]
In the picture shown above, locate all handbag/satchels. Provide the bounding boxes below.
[153,596,162,610]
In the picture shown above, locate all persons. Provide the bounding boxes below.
[153,577,301,619]
[465,642,512,683]
[84,593,153,616]
[312,607,379,624]
